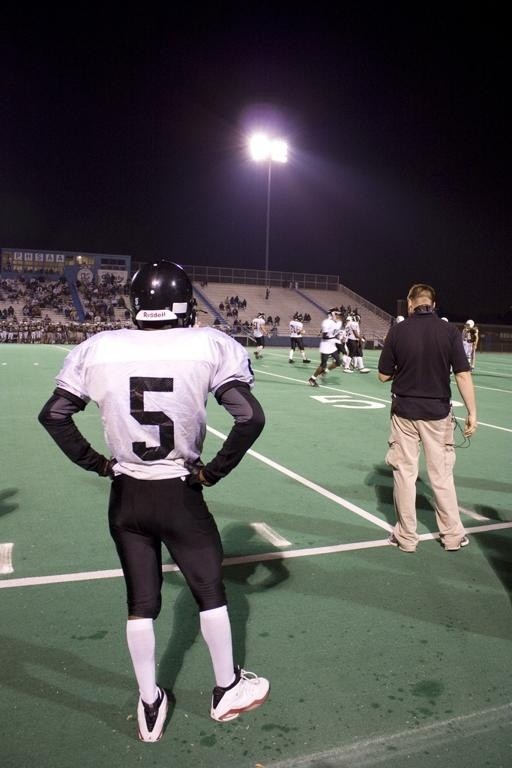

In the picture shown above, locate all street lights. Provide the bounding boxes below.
[247,131,291,286]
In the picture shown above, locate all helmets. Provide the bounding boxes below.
[123,260,198,328]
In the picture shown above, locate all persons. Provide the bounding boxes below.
[36,257,269,742]
[462,317,481,374]
[199,278,368,386]
[379,283,477,555]
[439,317,449,322]
[1,258,137,347]
[396,315,405,324]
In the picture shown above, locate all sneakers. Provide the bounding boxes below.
[138,684,173,746]
[211,666,270,721]
[445,534,470,551]
[386,532,417,553]
